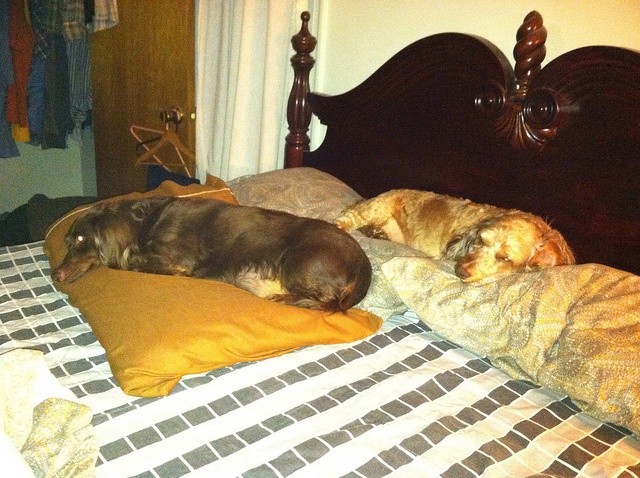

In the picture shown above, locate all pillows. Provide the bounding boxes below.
[226,167,454,318]
[382,260,640,440]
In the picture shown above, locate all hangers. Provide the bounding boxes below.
[130,108,198,167]
[133,107,191,174]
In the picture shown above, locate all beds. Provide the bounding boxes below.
[1,12,640,478]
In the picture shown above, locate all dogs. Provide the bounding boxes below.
[335,188,577,288]
[51,194,372,316]
